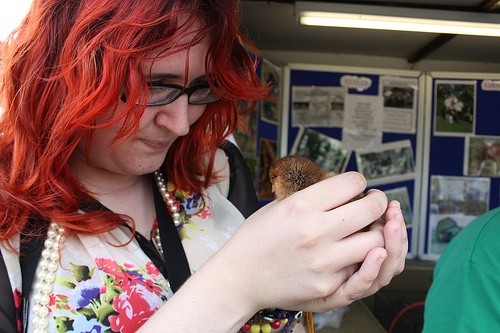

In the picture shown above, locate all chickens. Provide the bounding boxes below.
[269,155,372,233]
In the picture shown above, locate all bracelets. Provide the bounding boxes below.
[240,309,303,333]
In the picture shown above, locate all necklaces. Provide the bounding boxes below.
[32,168,182,333]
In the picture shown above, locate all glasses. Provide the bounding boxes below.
[119,74,227,106]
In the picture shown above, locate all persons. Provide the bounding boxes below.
[0,0,409,333]
[422,207,500,333]
